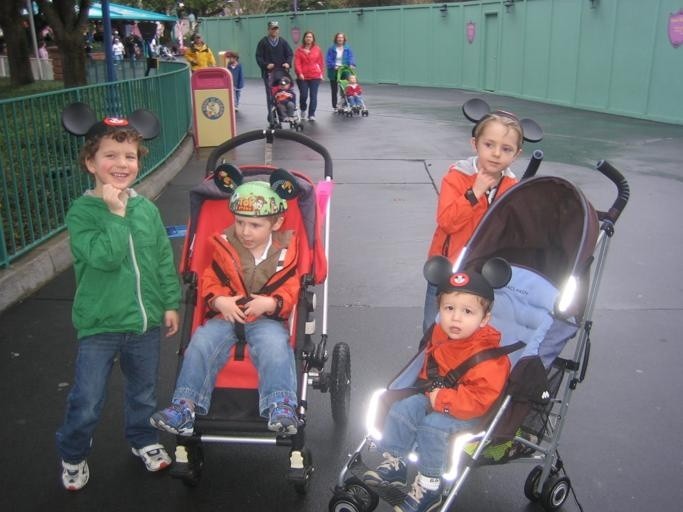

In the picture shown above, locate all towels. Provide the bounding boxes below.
[191,67,237,148]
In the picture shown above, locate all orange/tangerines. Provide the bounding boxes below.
[262,62,305,133]
[332,63,369,118]
[162,119,358,500]
[323,147,632,512]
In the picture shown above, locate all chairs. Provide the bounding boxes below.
[282,116,295,122]
[300,109,316,121]
[149,398,197,437]
[266,398,299,435]
[131,441,172,473]
[392,472,443,512]
[61,456,90,492]
[361,452,409,490]
[333,104,362,114]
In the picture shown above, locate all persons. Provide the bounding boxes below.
[148,162,301,440]
[254,20,293,129]
[291,30,324,123]
[417,97,546,334]
[225,52,243,115]
[51,101,183,493]
[356,254,512,512]
[183,34,217,74]
[345,73,364,113]
[272,76,299,124]
[324,31,357,112]
[36,40,48,65]
[110,28,176,63]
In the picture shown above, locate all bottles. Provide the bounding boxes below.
[212,162,301,219]
[462,97,544,146]
[60,101,161,141]
[267,19,280,29]
[422,254,513,302]
